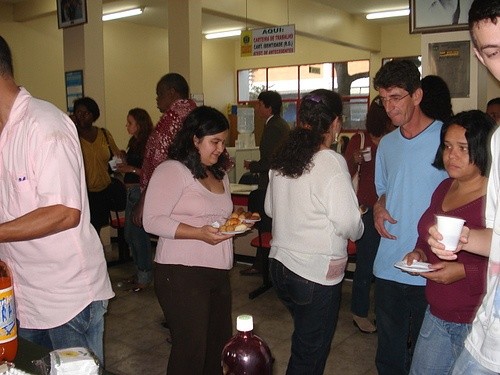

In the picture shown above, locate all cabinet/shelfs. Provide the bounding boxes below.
[226,148,261,185]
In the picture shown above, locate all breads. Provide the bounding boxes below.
[219,206,260,232]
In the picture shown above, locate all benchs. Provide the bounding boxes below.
[249,233,356,300]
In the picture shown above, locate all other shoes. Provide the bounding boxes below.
[240,267,261,276]
[121,275,136,286]
[129,284,150,294]
[352,314,377,333]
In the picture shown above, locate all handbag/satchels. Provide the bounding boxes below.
[352,130,364,194]
[110,175,127,211]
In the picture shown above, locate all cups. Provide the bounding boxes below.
[361,149,371,162]
[108,159,122,171]
[246,160,252,163]
[435,215,466,251]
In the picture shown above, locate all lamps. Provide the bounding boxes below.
[102,7,145,21]
[206,29,243,40]
[366,9,410,20]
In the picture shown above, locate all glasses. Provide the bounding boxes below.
[374,93,410,107]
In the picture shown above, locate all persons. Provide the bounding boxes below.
[372,60,451,375]
[143,105,237,375]
[67,96,124,248]
[340,135,349,154]
[401,109,491,375]
[112,108,156,295]
[418,74,457,123]
[0,35,114,374]
[265,89,364,375]
[344,96,400,333]
[132,72,235,237]
[238,91,290,276]
[485,96,500,129]
[238,159,262,185]
[427,0,500,375]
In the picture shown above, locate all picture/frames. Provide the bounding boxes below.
[409,0,473,34]
[57,0,87,29]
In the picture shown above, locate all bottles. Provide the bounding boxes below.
[0,258,18,365]
[221,314,272,375]
[237,108,254,134]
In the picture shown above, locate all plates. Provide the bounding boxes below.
[225,217,261,223]
[393,261,437,273]
[214,226,251,234]
